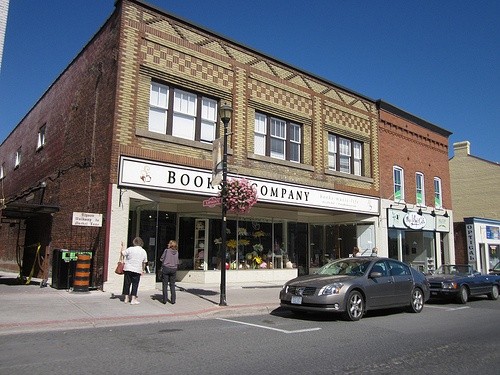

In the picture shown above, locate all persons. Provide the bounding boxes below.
[370,248,379,257]
[160,240,179,304]
[352,247,361,256]
[120,237,148,304]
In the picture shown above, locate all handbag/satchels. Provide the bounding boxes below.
[114,253,125,275]
[158,266,163,280]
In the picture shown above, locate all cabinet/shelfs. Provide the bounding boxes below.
[194,219,209,272]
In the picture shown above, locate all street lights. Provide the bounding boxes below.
[218,102,234,306]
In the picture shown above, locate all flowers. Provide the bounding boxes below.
[223,177,258,212]
[213,227,265,264]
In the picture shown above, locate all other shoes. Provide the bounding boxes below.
[172,302,176,304]
[163,302,166,304]
[131,300,140,305]
[124,299,130,303]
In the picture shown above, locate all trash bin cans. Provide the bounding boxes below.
[52,249,93,290]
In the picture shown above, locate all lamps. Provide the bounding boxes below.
[422,207,437,217]
[407,202,422,216]
[436,209,450,218]
[390,201,408,213]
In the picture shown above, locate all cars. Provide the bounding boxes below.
[280,255,430,321]
[425,261,500,304]
